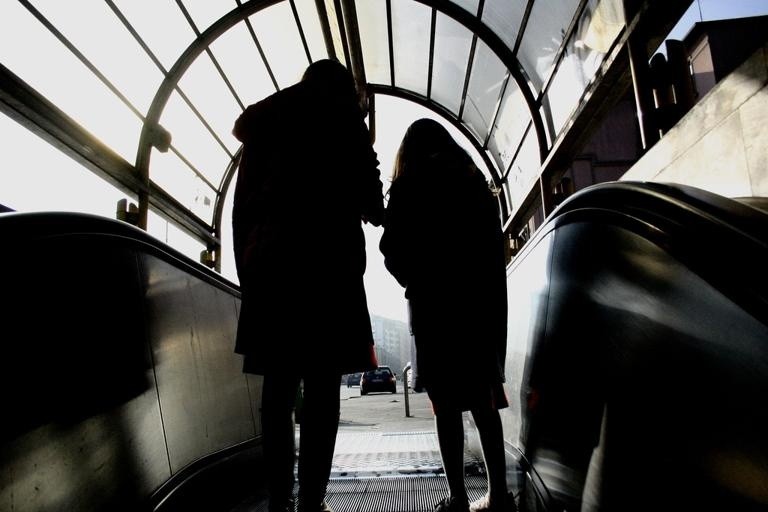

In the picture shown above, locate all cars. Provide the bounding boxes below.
[360,365,397,395]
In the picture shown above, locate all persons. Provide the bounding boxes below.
[231,58,387,512]
[376,117,517,512]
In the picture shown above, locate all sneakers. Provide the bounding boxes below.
[434,495,469,512]
[269,493,331,512]
[470,491,517,512]
